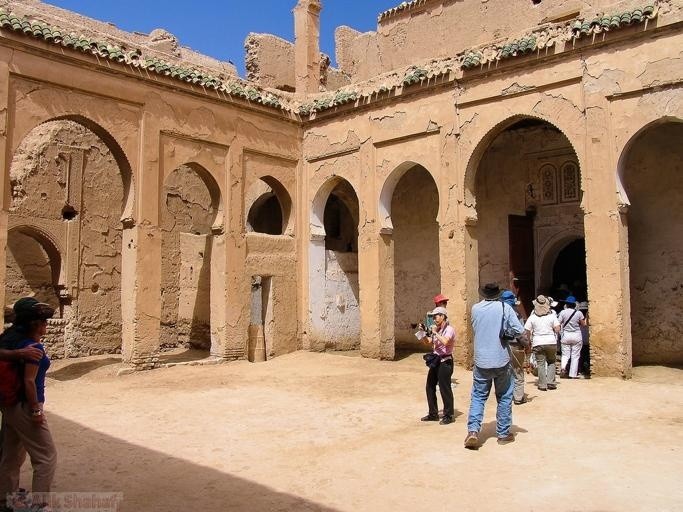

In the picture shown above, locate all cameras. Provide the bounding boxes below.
[422,325,433,337]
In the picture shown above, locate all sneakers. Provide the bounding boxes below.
[438,414,455,425]
[547,383,557,389]
[463,431,481,448]
[12,487,56,512]
[538,384,546,391]
[421,414,439,422]
[559,369,566,379]
[497,432,516,445]
[513,396,527,404]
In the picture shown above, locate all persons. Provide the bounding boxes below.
[0,302,59,512]
[0,297,44,363]
[418,270,588,448]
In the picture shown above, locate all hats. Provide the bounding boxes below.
[434,293,450,304]
[12,297,56,320]
[427,306,448,318]
[514,297,521,306]
[499,290,517,305]
[560,296,588,310]
[532,295,559,316]
[478,280,504,301]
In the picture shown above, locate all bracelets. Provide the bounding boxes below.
[31,406,43,417]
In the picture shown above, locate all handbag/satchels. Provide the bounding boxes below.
[422,352,440,370]
